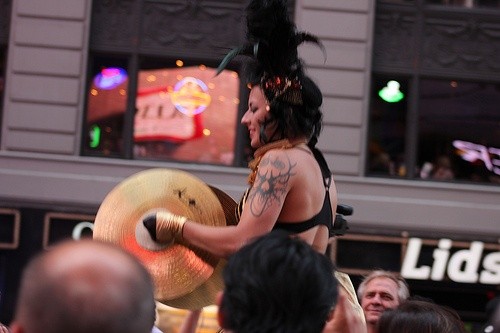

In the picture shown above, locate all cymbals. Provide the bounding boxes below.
[92,167,239,311]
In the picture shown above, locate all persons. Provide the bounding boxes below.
[7,240,158,333]
[356,270,408,332]
[143,69,337,256]
[375,300,465,333]
[213,232,340,333]
